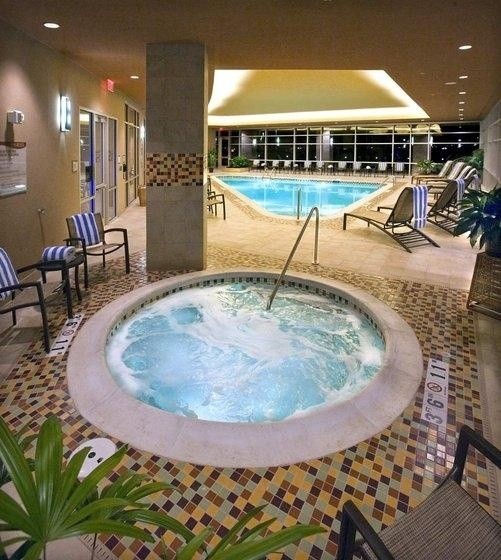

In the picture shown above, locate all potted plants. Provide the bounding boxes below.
[207,149,218,173]
[448,183,500,319]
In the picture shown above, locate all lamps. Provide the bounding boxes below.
[57,96,73,134]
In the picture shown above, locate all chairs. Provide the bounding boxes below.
[206,176,227,220]
[333,422,500,559]
[339,160,481,260]
[249,158,407,180]
[0,211,132,354]
